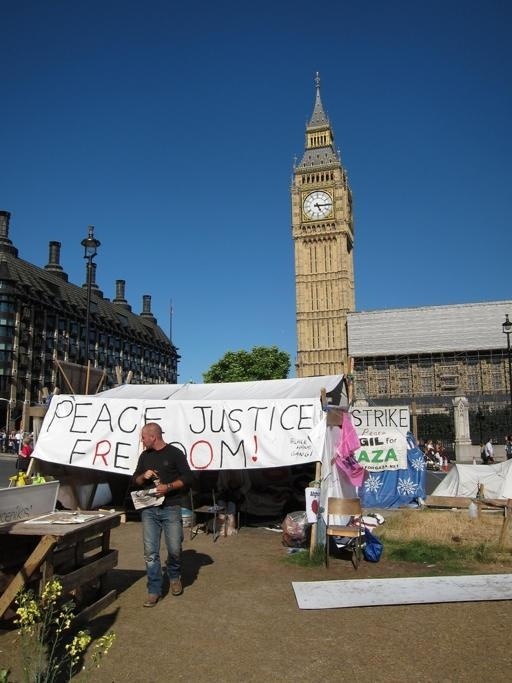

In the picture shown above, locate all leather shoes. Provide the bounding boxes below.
[144,592,160,607]
[170,576,183,596]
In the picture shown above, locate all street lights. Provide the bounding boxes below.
[81,224,102,364]
[501,312,512,413]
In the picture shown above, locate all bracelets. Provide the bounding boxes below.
[168,483,173,492]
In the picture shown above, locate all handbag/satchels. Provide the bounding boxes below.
[16,458,31,469]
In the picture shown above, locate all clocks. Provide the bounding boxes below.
[302,190,333,221]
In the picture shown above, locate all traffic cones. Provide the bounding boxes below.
[442,456,450,473]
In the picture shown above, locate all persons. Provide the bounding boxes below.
[425,436,448,471]
[216,470,252,513]
[0,427,33,472]
[128,422,194,608]
[479,434,512,465]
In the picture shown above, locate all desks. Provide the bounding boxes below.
[0,510,124,628]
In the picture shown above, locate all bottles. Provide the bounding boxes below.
[196,519,208,534]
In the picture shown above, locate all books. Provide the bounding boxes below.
[130,488,165,510]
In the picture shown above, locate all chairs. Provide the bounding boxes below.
[189,485,227,541]
[326,496,363,567]
